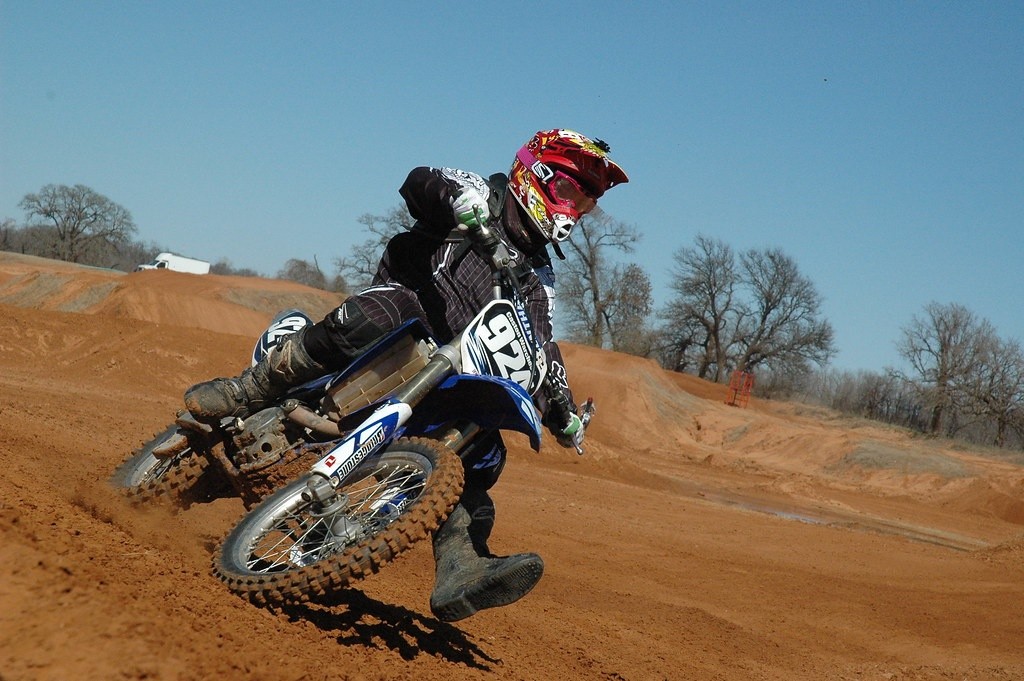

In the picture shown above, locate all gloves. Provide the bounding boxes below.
[450,186,490,231]
[556,412,585,448]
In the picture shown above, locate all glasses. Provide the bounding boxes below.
[544,170,598,220]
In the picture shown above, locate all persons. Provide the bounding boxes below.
[580,397,596,423]
[183,128,629,623]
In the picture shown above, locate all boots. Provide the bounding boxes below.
[430,502,546,623]
[184,326,334,425]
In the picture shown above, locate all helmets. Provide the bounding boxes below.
[508,128,629,242]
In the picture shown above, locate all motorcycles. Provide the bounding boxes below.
[110,182,596,613]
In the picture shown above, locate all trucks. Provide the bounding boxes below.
[136,253,211,277]
